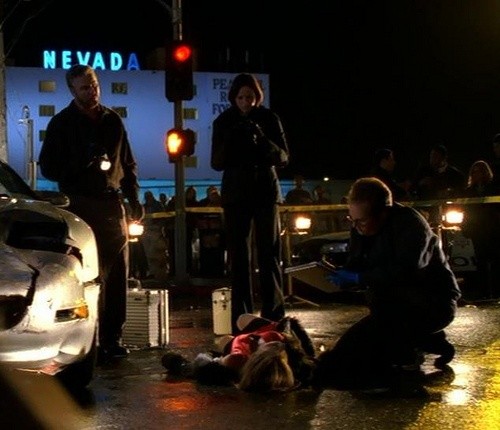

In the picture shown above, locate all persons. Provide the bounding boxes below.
[159,313,314,394]
[39,65,145,369]
[210,73,290,323]
[143,133,500,287]
[311,176,463,398]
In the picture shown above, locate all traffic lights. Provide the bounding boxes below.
[165,128,187,163]
[164,39,195,101]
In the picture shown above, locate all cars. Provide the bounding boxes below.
[0,157,103,388]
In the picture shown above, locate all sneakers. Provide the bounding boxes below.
[429,331,455,367]
[100,341,128,358]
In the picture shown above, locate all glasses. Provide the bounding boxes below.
[346,216,371,225]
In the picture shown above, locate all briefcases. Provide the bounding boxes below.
[119,277,170,350]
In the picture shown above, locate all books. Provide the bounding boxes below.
[284,261,345,292]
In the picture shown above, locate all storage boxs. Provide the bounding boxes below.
[211,286,232,335]
[119,277,170,349]
